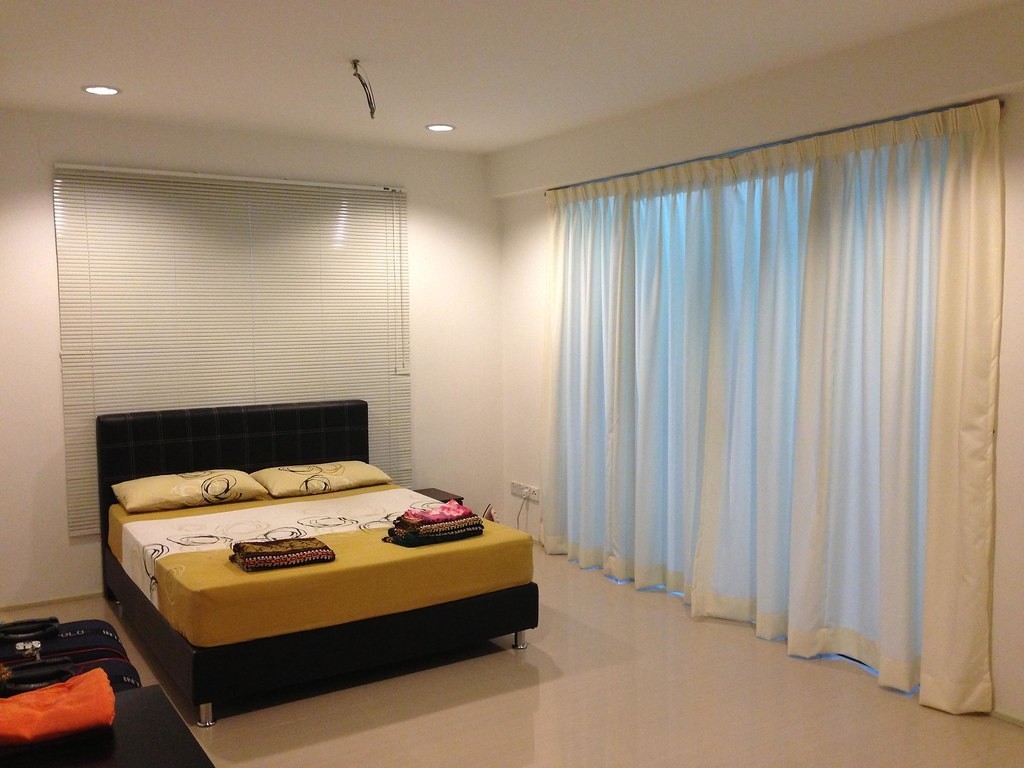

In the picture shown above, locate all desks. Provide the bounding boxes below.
[0,683,216,768]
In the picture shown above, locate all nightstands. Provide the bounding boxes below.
[413,487,464,506]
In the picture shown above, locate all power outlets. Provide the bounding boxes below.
[510,480,539,502]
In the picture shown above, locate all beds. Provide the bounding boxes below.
[95,399,540,728]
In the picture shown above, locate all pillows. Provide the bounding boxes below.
[250,460,393,498]
[111,468,269,514]
